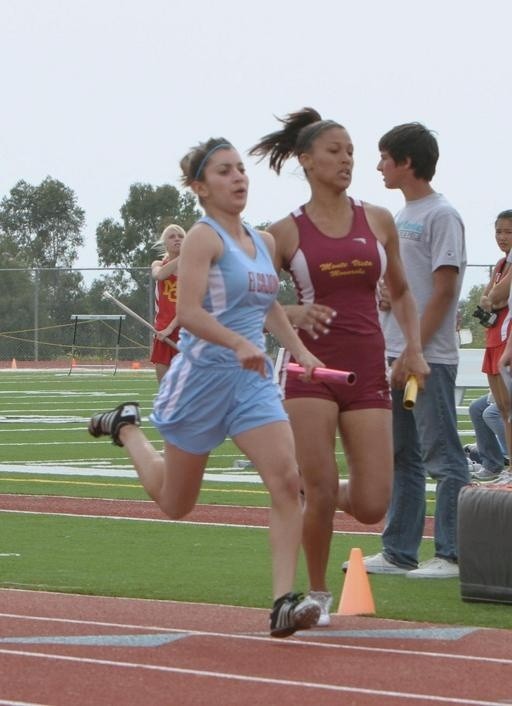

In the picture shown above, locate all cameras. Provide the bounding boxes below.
[472,305,499,327]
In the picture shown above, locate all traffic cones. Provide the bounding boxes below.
[72,358,79,368]
[330,546,381,620]
[11,358,19,368]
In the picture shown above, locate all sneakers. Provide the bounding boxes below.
[88,402,143,447]
[309,590,333,627]
[342,553,409,576]
[269,593,321,638]
[407,557,459,579]
[464,442,512,488]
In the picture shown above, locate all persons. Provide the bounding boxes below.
[145,222,189,385]
[476,207,512,486]
[340,122,475,582]
[496,330,512,380]
[86,134,332,637]
[247,104,433,629]
[468,388,510,482]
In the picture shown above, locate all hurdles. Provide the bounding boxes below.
[67,314,127,377]
